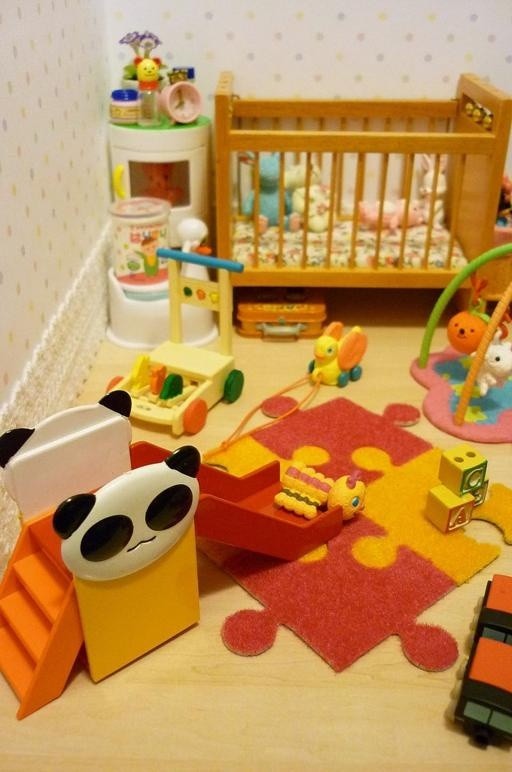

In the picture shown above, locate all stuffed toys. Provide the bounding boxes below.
[239,147,452,238]
[495,179,511,233]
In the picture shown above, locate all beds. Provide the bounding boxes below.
[212,70,510,306]
